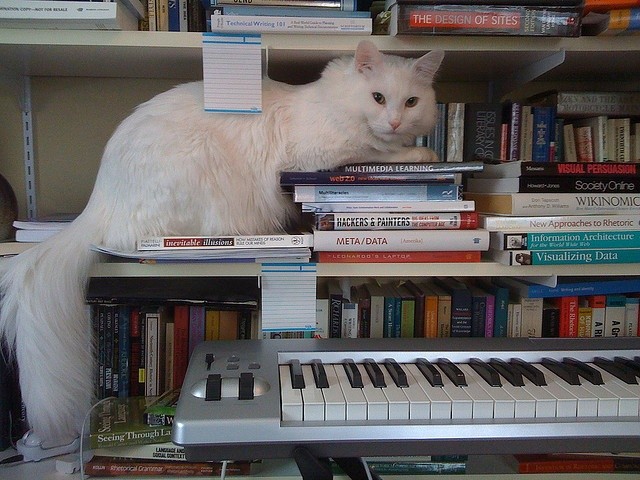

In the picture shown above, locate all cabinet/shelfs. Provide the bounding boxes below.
[0,30,639,480]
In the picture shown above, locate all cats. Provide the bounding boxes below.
[1,40,444,441]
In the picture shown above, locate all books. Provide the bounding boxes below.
[15,230,63,242]
[134,160,640,262]
[1,1,639,39]
[13,213,82,230]
[414,92,640,165]
[87,297,258,480]
[251,279,640,341]
[362,452,639,477]
